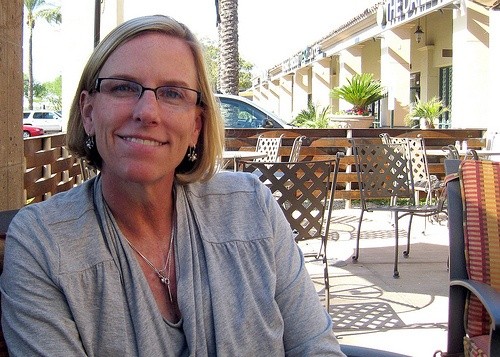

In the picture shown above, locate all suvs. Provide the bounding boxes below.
[23,110,62,132]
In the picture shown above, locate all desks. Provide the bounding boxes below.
[413,149,500,160]
[218,151,268,171]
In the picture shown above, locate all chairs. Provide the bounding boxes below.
[0,132,500,357]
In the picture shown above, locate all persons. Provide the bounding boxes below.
[0,14,346,357]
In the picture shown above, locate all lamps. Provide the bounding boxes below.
[414,25,424,43]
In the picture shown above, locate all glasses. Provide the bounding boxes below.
[94,76,201,112]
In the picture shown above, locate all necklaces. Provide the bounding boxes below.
[123,221,176,307]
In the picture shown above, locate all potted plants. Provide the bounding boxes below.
[330,73,386,129]
[403,93,448,129]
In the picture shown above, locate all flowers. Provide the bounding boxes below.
[345,107,369,116]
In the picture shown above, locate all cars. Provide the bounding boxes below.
[214,94,296,130]
[22,125,45,139]
